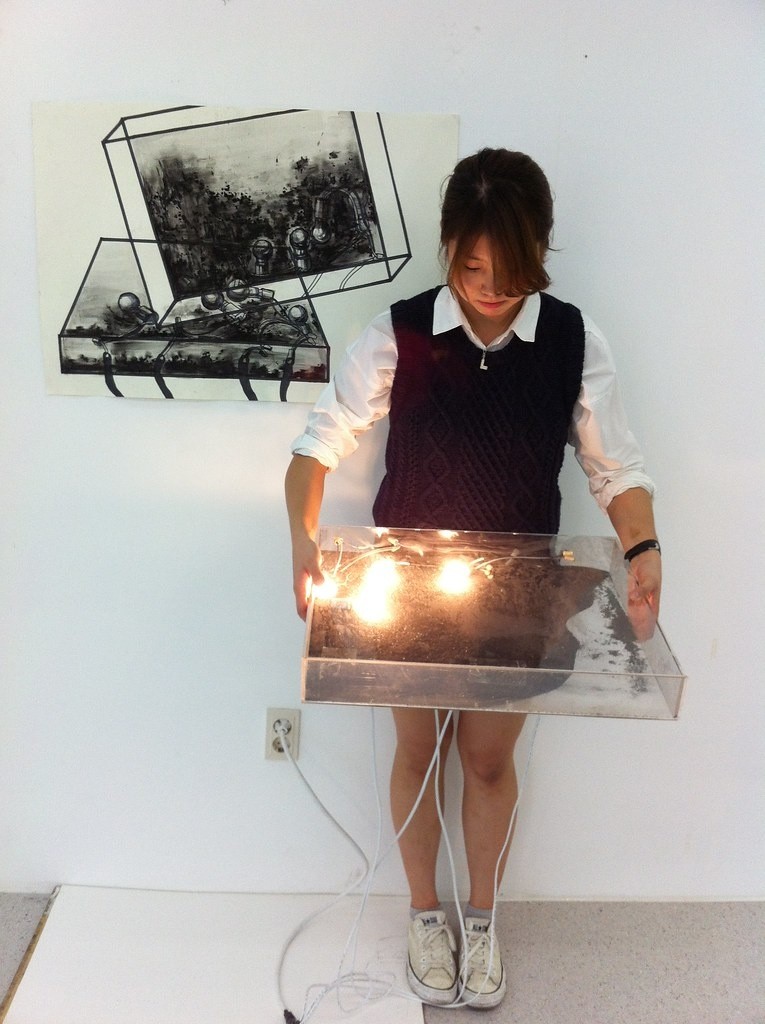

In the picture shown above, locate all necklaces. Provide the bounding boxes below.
[458,297,522,371]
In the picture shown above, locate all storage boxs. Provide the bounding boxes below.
[300,523,684,722]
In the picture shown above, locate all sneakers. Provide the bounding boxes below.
[457,917,506,1009]
[406,910,458,1005]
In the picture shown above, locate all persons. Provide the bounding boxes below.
[285,147,662,1010]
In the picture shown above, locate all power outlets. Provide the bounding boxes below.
[264,709,301,762]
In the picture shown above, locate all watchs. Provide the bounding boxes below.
[623,539,661,563]
